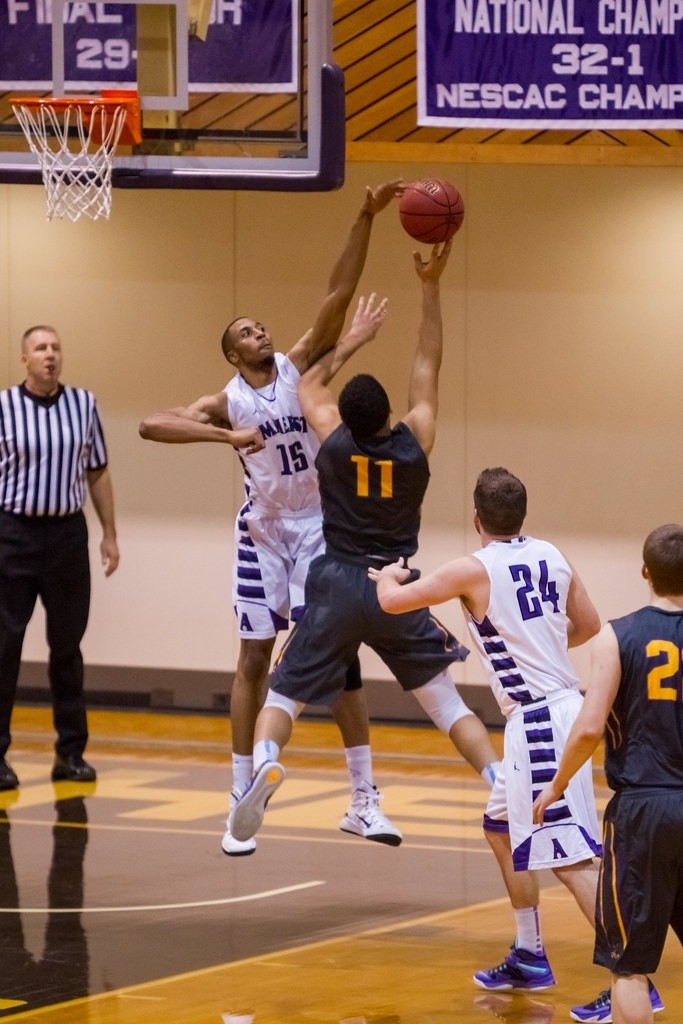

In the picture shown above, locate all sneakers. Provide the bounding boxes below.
[472,934,557,994]
[221,817,256,856]
[227,759,287,842]
[569,976,665,1024]
[339,781,403,847]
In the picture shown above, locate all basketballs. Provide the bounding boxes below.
[399,176,465,245]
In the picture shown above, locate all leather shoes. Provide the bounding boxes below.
[51,759,97,782]
[0,757,20,791]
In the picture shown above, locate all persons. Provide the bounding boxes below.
[531,523,683,1022]
[367,464,666,1022]
[0,324,120,790]
[141,176,404,856]
[228,238,503,842]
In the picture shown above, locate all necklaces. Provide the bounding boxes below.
[235,364,279,402]
